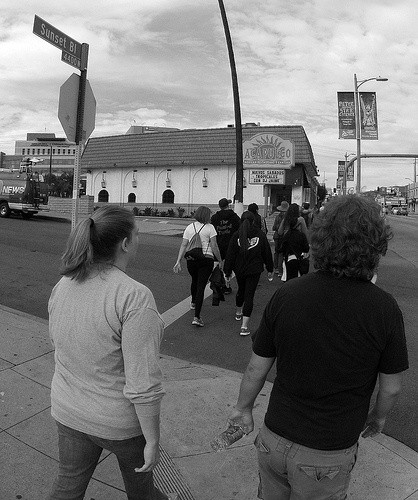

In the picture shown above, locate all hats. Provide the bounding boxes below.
[218,198,232,208]
[276,201,289,211]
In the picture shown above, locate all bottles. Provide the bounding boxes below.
[212,425,242,452]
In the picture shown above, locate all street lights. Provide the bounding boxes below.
[352,73,388,195]
[344,152,356,196]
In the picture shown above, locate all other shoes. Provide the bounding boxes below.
[278,273,282,277]
[167,493,179,500]
[274,268,280,273]
[225,287,232,294]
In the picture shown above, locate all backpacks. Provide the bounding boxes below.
[183,222,210,261]
[300,211,311,229]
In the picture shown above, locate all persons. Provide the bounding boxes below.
[300,203,312,228]
[272,201,290,278]
[360,94,376,130]
[49,204,179,500]
[223,211,274,336]
[230,193,409,500]
[277,203,309,282]
[210,198,240,294]
[313,203,324,212]
[248,203,268,234]
[173,205,224,326]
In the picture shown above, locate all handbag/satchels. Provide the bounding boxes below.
[275,234,285,252]
[299,256,309,276]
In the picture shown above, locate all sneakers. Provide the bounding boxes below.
[192,317,205,326]
[239,328,250,335]
[235,309,243,320]
[190,301,195,309]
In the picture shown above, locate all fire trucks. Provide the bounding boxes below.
[0,168,50,220]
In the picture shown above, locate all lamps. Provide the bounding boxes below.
[131,173,137,187]
[202,171,207,187]
[166,172,171,187]
[243,168,247,188]
[101,174,106,188]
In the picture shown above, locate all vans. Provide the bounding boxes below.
[391,207,407,216]
